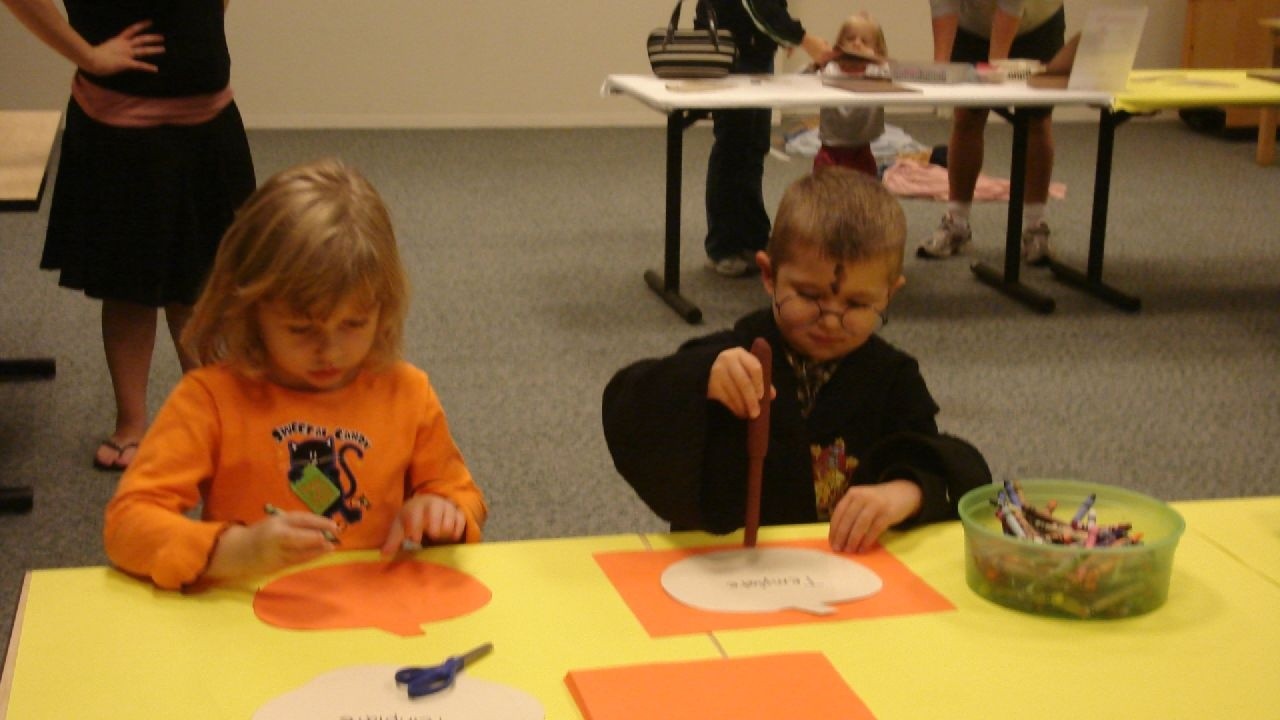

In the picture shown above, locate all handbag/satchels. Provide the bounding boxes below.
[648,0,734,78]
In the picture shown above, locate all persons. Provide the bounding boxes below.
[600,164,993,556]
[916,0,1067,269]
[104,158,487,592]
[694,0,829,276]
[802,11,892,176]
[2,0,260,472]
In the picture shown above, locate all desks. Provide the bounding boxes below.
[1045,68,1280,312]
[601,73,1114,322]
[0,497,1280,720]
[0,109,65,511]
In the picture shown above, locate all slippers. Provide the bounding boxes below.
[94,438,138,473]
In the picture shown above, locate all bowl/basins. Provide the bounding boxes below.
[956,478,1188,620]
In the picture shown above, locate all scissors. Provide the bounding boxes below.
[394,641,494,697]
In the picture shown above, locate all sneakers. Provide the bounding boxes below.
[716,257,747,277]
[1021,223,1050,266]
[916,216,972,259]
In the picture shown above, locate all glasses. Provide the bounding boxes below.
[771,268,891,337]
